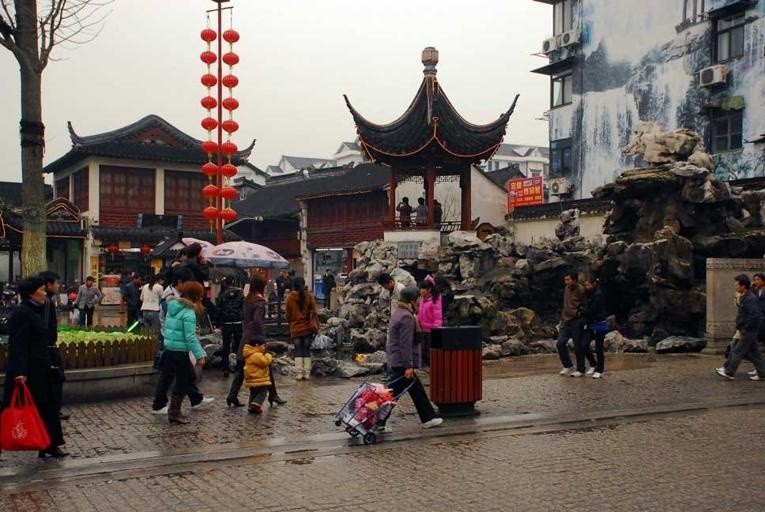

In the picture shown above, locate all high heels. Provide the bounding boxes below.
[37,446,70,459]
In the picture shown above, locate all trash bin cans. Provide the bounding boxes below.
[314,279,325,300]
[429,326,483,418]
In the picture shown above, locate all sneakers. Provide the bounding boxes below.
[560,366,574,375]
[152,407,169,415]
[381,426,393,433]
[748,369,764,380]
[593,371,601,379]
[571,371,585,378]
[248,402,264,414]
[715,367,735,380]
[587,367,595,375]
[193,396,214,409]
[422,415,444,429]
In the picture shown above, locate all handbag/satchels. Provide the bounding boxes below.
[0,404,51,450]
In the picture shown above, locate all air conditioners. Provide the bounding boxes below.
[560,29,580,48]
[699,63,727,88]
[541,37,558,54]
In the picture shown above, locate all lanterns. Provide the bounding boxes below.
[200,26,240,226]
[108,243,119,261]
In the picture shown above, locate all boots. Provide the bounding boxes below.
[268,371,287,407]
[168,392,190,423]
[227,373,244,408]
[303,357,312,381]
[294,357,303,380]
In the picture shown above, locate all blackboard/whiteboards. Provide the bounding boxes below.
[243,283,250,297]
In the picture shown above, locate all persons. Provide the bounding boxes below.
[412,197,428,226]
[39,270,72,421]
[578,278,608,379]
[377,272,406,314]
[396,196,412,226]
[555,270,586,378]
[433,198,442,227]
[376,287,444,434]
[0,276,70,459]
[714,272,765,382]
[117,242,336,425]
[72,276,102,328]
[418,274,443,334]
